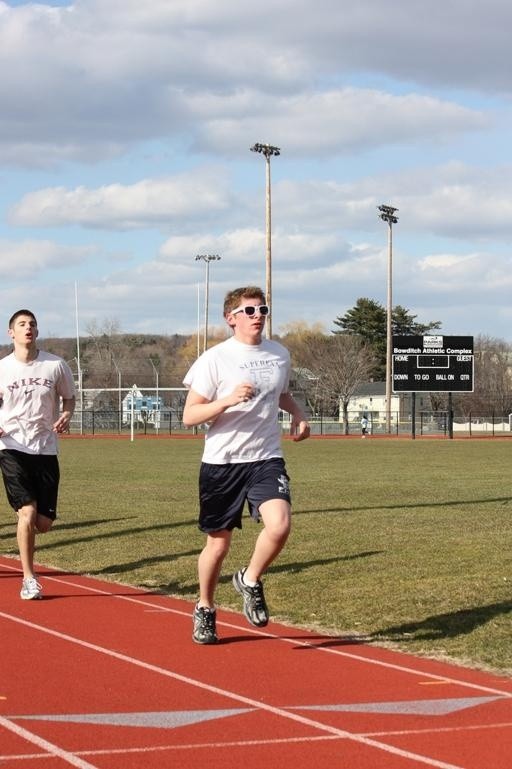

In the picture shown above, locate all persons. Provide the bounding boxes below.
[182,286,311,646]
[0,308,77,601]
[360,415,371,438]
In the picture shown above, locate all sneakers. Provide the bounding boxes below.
[20,575,43,600]
[232,566,269,628]
[191,599,219,645]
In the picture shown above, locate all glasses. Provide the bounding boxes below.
[231,304,269,316]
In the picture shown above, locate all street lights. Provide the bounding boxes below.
[251,143,282,341]
[376,204,400,434]
[195,253,221,352]
